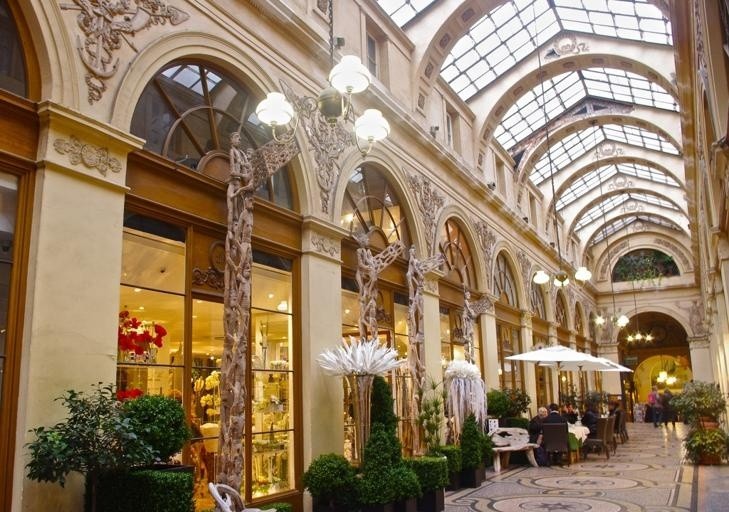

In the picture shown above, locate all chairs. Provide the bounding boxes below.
[208,482,277,512]
[486,410,629,471]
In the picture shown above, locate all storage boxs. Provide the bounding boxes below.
[254,383,280,402]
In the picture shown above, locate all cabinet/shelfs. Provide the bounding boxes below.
[118,365,148,401]
[169,353,208,436]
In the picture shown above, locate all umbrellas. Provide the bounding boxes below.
[538,353,611,401]
[594,357,635,393]
[504,345,609,406]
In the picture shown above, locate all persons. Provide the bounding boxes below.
[662,388,676,428]
[215,129,255,495]
[355,233,378,338]
[529,400,624,466]
[463,292,475,365]
[685,379,693,392]
[406,245,426,382]
[648,385,665,428]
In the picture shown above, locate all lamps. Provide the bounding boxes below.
[254,0,391,170]
[622,218,654,342]
[588,119,628,329]
[531,0,591,289]
[656,343,677,386]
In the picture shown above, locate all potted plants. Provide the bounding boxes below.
[301,404,493,512]
[484,388,512,432]
[668,379,729,466]
[122,393,194,479]
[503,386,532,417]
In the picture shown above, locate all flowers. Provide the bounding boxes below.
[118,310,166,354]
